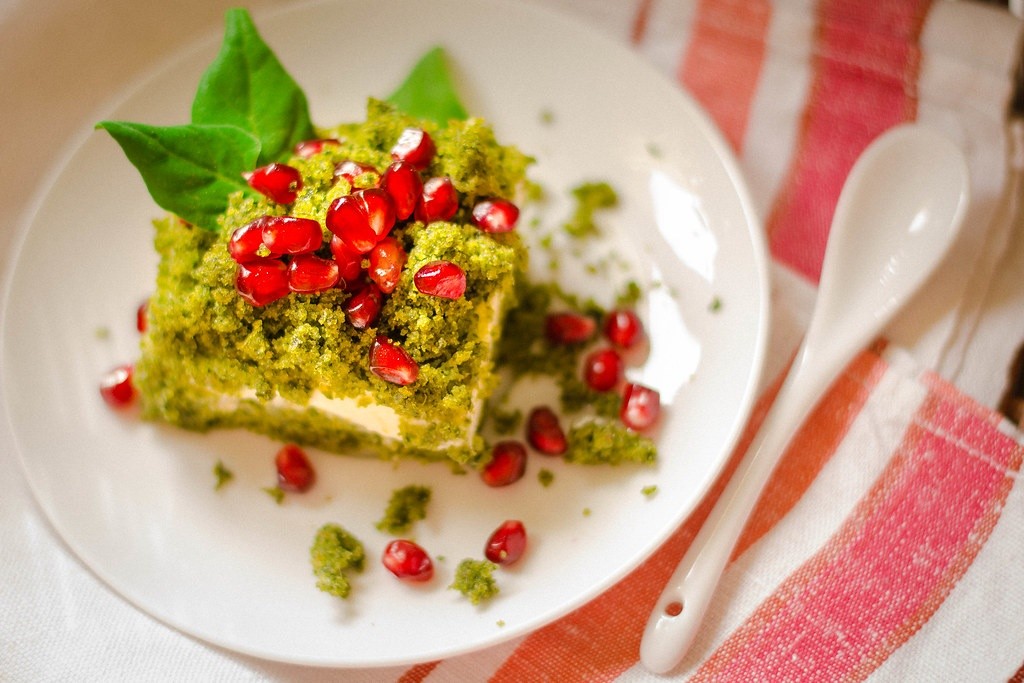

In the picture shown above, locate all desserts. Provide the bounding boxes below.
[140,120,522,456]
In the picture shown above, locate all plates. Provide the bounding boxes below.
[0,0,772,670]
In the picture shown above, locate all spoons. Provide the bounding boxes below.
[640,123,971,675]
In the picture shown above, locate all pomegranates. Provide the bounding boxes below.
[586,350,620,390]
[548,314,597,340]
[605,312,642,345]
[276,445,316,491]
[382,539,433,581]
[483,442,527,486]
[485,520,526,564]
[621,384,660,429]
[100,368,135,406]
[527,406,568,453]
